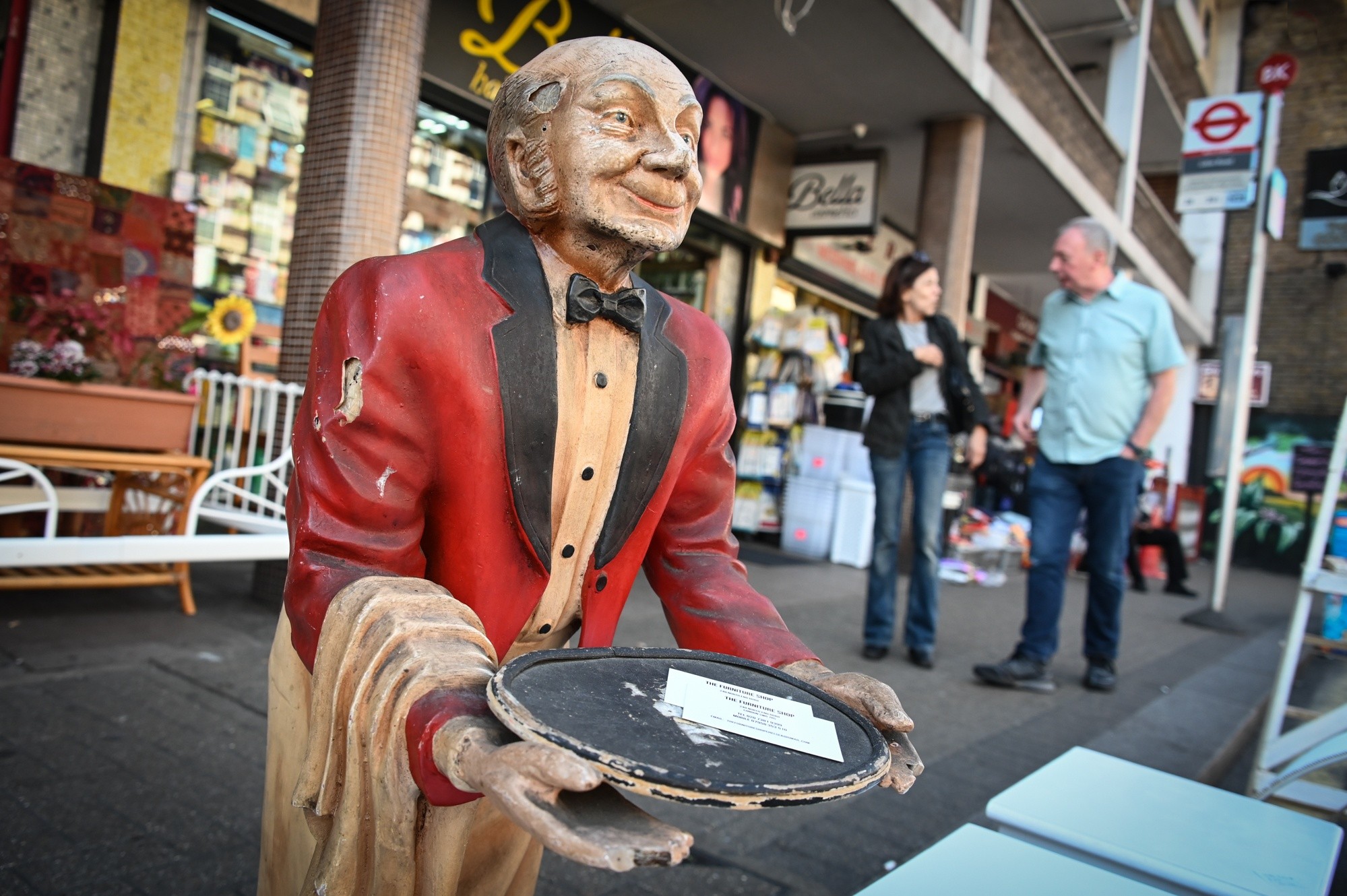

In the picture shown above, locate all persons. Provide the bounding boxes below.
[971,217,1202,694]
[853,252,989,670]
[257,35,924,895]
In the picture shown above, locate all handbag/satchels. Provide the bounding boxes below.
[938,362,979,436]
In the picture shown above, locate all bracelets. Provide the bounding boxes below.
[1127,441,1152,463]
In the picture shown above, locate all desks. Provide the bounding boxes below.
[0,442,217,615]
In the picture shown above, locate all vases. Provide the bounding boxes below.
[0,373,202,455]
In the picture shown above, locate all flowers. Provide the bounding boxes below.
[3,256,259,393]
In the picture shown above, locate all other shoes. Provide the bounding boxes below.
[864,645,888,659]
[970,654,1049,689]
[908,647,935,668]
[1166,582,1198,597]
[1083,660,1119,691]
